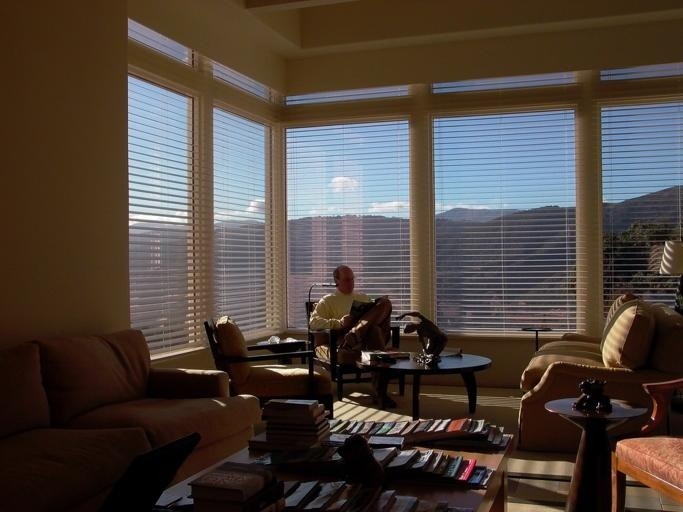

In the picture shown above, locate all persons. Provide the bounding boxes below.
[308,265,398,409]
[395,310,448,364]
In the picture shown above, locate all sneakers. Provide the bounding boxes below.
[371,394,398,408]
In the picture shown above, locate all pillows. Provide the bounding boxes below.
[600,293,655,372]
[1,326,152,438]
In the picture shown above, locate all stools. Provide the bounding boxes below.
[522,327,553,353]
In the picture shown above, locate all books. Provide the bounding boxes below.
[186,397,511,511]
[360,349,409,360]
[348,294,389,325]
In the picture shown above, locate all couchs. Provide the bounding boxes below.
[517,304,682,454]
[0,366,260,512]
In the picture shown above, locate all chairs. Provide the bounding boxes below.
[610,375,683,512]
[203,282,400,419]
